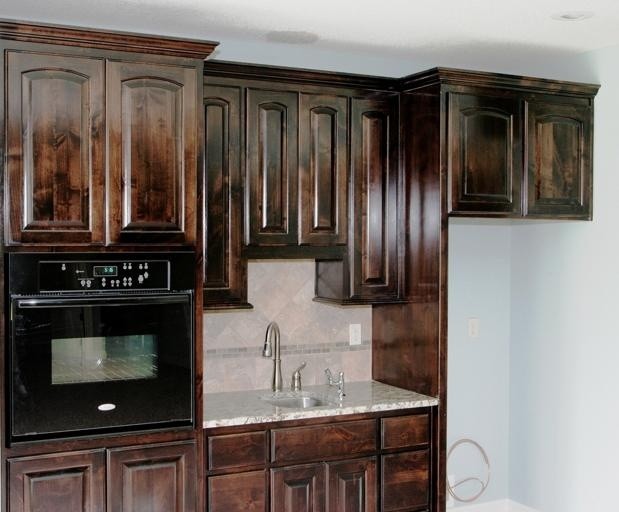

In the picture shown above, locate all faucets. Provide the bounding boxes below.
[261,320,283,392]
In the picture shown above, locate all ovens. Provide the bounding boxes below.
[7,290,195,445]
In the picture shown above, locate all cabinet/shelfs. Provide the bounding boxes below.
[0,17,220,254]
[206,412,435,512]
[202,60,408,310]
[437,67,602,221]
[6,438,200,512]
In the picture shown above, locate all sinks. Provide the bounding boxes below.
[264,393,327,411]
